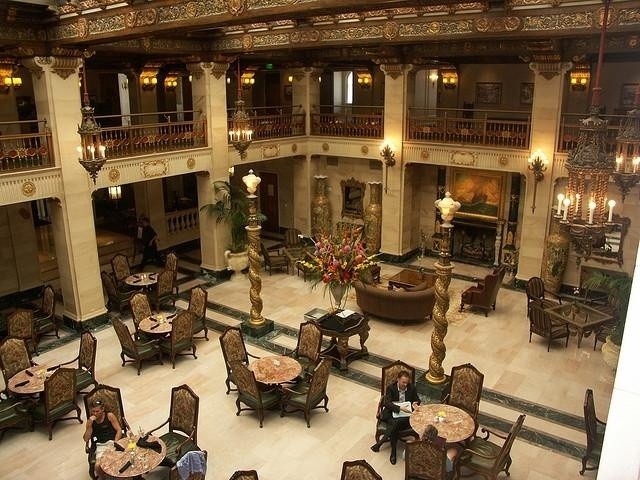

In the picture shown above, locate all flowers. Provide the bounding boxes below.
[295,217,386,299]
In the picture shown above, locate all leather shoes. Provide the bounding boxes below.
[370,443,379,452]
[389,453,397,466]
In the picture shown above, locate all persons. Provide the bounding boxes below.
[136,218,165,272]
[83,400,122,480]
[371,371,421,464]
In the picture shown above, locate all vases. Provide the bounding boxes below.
[365,180,382,256]
[311,175,331,236]
[328,284,348,315]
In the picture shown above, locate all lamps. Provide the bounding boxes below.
[380,145,396,167]
[77,58,108,186]
[554,2,625,268]
[612,85,640,204]
[167,80,177,89]
[527,150,549,182]
[227,52,252,161]
[288,76,293,81]
[357,77,369,86]
[142,77,158,90]
[0,68,22,94]
[242,77,256,86]
[443,76,454,88]
[571,78,588,90]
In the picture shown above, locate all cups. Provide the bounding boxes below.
[141,458,149,468]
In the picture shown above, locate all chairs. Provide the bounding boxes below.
[149,269,176,315]
[146,383,199,466]
[363,265,381,284]
[297,257,317,282]
[528,305,570,352]
[184,285,209,341]
[6,308,40,356]
[229,470,258,480]
[440,364,484,448]
[340,459,381,480]
[29,368,84,441]
[83,385,133,480]
[375,359,417,444]
[46,329,98,395]
[112,317,163,375]
[110,253,132,288]
[454,413,528,480]
[0,390,34,442]
[284,228,307,248]
[165,251,180,298]
[261,242,289,276]
[280,358,333,428]
[281,319,323,372]
[593,323,616,350]
[0,335,41,390]
[404,441,447,480]
[588,289,621,317]
[457,263,509,317]
[227,359,282,428]
[526,276,561,308]
[580,389,606,473]
[219,326,261,394]
[101,271,136,316]
[129,292,159,331]
[171,449,207,479]
[159,310,197,369]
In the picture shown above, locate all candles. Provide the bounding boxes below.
[101,146,106,158]
[575,194,579,213]
[99,145,101,155]
[236,131,240,141]
[588,202,596,224]
[91,147,96,160]
[86,145,90,156]
[563,198,571,220]
[249,130,252,140]
[77,146,82,160]
[246,131,249,140]
[607,200,617,222]
[556,193,564,214]
[228,131,233,141]
[242,130,245,140]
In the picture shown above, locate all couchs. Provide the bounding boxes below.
[354,270,438,322]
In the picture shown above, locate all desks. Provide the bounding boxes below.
[282,247,316,275]
[99,434,167,480]
[125,272,160,289]
[545,300,611,351]
[8,365,59,421]
[409,403,481,443]
[139,314,178,338]
[248,356,303,385]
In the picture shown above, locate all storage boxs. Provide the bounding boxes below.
[34,284,60,343]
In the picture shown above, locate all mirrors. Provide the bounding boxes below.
[585,210,631,267]
[340,176,366,221]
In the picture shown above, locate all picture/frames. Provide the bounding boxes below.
[381,81,385,100]
[520,82,535,105]
[475,81,502,105]
[448,168,508,224]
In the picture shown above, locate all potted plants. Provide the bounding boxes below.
[581,273,633,373]
[198,180,269,281]
